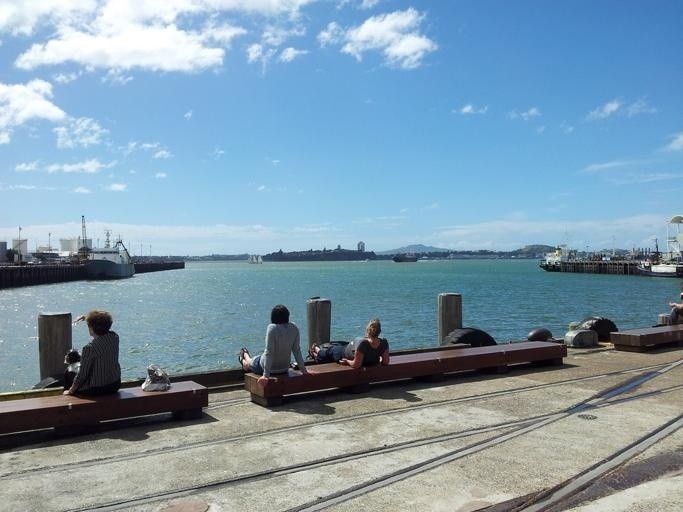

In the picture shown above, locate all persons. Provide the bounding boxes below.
[238,304,317,385]
[62,348,81,388]
[306,318,389,368]
[62,310,121,397]
[665,291,682,326]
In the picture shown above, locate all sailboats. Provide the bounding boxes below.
[245,254,263,264]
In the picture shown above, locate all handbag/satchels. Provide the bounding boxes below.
[142,364,170,391]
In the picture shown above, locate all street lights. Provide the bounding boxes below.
[17,224,21,266]
[48,232,51,265]
[96,238,151,259]
[586,245,589,257]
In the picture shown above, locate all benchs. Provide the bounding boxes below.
[243,340,567,408]
[609,324,683,353]
[0,380,208,435]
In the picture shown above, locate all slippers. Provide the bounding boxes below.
[239,348,249,371]
[309,343,318,358]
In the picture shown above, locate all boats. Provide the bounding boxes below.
[84,230,136,280]
[391,253,417,263]
[635,261,683,278]
[538,246,562,271]
